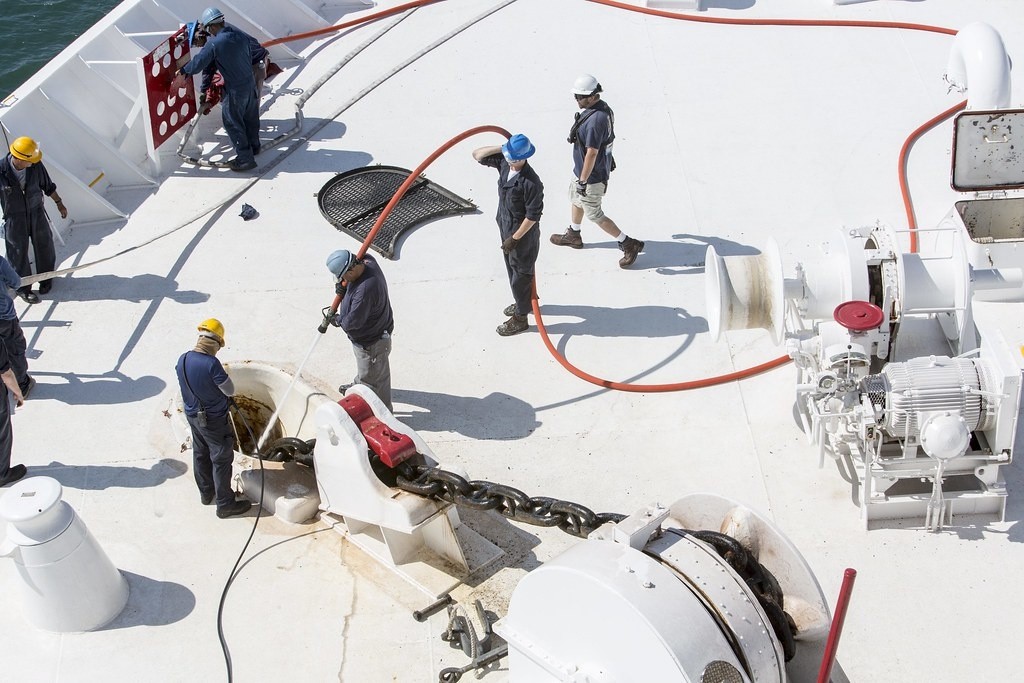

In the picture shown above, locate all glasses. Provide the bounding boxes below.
[31,142,40,155]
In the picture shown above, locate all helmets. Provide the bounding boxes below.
[201,8,224,27]
[326,250,352,283]
[571,74,603,96]
[184,19,199,49]
[10,136,42,163]
[197,318,225,347]
[501,134,536,162]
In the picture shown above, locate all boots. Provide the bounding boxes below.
[550,225,584,249]
[618,235,644,268]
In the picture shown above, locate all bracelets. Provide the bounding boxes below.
[55,198,61,203]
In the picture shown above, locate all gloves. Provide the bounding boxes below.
[329,314,340,327]
[576,180,587,197]
[335,283,347,297]
[501,235,520,254]
[199,95,211,116]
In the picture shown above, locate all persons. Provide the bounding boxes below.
[549,76,644,268]
[175,318,252,517]
[325,249,394,413]
[471,134,543,335]
[171,8,271,169]
[0,256,37,484]
[0,137,67,304]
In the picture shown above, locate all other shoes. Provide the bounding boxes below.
[15,290,38,304]
[503,302,516,316]
[253,143,261,155]
[496,312,529,336]
[216,500,251,518]
[22,378,35,399]
[200,489,215,505]
[0,464,27,487]
[229,156,257,171]
[39,279,52,294]
